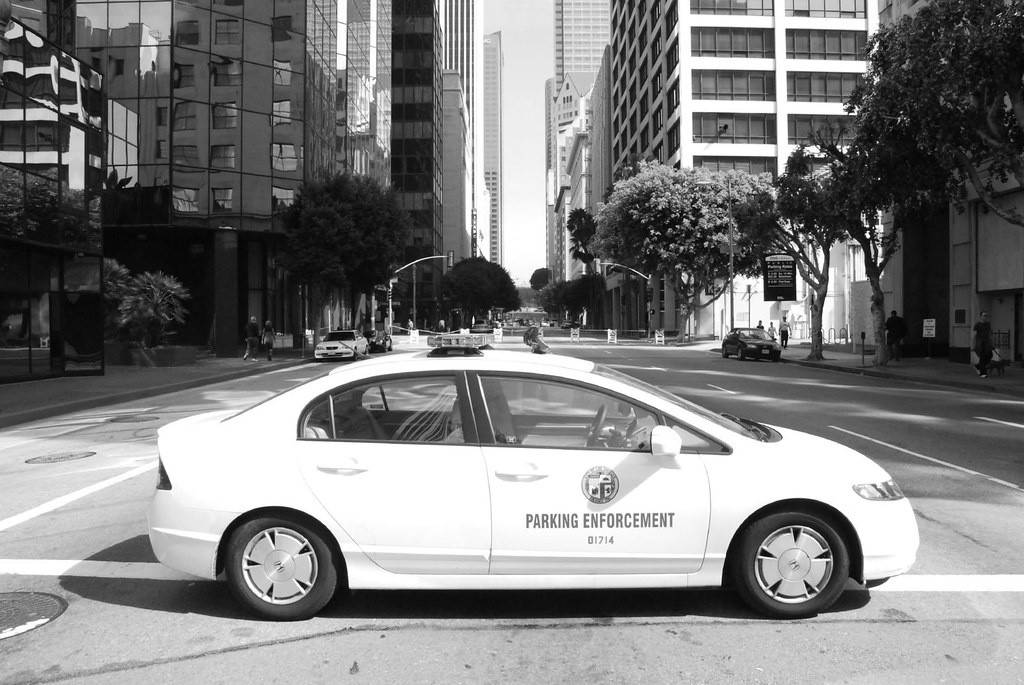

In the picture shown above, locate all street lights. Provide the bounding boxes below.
[696,179,734,330]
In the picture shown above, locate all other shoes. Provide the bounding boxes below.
[974,365,980,374]
[252,358,258,362]
[243,355,246,359]
[980,374,987,378]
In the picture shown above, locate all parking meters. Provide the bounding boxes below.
[860,332,865,364]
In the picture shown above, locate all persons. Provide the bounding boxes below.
[407,319,413,335]
[779,317,792,350]
[261,320,276,361]
[757,320,764,329]
[243,316,260,361]
[768,322,777,341]
[970,310,995,378]
[884,310,909,362]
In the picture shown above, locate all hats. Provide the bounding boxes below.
[265,321,271,327]
[250,316,257,321]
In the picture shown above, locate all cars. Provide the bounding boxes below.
[360,330,393,353]
[314,329,369,361]
[561,322,571,330]
[147,344,920,619]
[721,328,782,362]
[533,307,544,312]
[539,318,550,327]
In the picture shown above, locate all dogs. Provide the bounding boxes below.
[986,359,1011,376]
